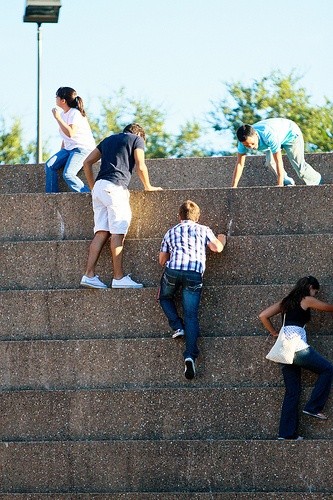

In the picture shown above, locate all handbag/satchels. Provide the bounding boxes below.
[265,312,309,364]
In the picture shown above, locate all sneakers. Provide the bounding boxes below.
[111,275,143,288]
[184,357,195,380]
[79,274,106,289]
[172,329,183,338]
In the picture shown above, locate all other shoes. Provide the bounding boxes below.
[277,436,305,439]
[303,409,328,420]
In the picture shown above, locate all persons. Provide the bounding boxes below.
[80,123,164,288]
[259,275,333,441]
[158,200,227,380]
[231,117,322,187]
[45,86,96,193]
